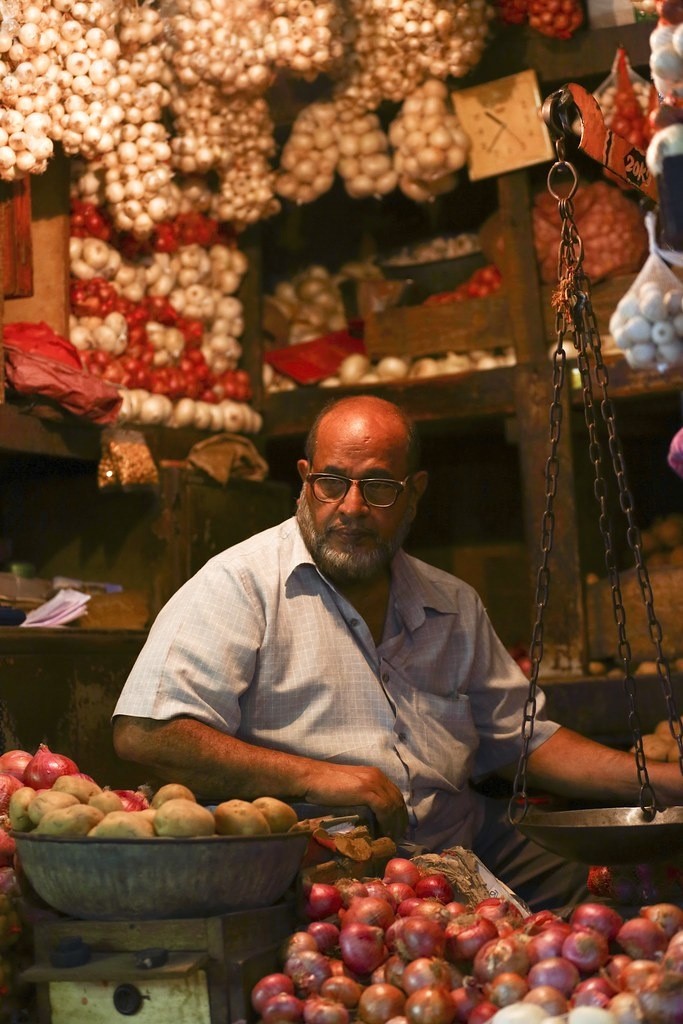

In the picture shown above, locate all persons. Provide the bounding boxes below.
[111,393,683,929]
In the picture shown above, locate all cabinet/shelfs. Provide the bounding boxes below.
[0,19,683,793]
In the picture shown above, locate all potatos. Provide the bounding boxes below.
[9,777,298,840]
[0,891,43,1024]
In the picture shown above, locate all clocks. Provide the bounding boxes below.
[451,69,555,183]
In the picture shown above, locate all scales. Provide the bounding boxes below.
[503,83,683,863]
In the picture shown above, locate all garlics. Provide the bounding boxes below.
[0,0,683,435]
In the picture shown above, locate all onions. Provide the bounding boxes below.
[248,858,682,1024]
[1,743,150,895]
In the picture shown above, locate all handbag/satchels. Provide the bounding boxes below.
[608,208,683,370]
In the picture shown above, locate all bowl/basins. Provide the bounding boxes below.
[8,826,314,919]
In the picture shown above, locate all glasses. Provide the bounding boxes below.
[305,473,412,509]
